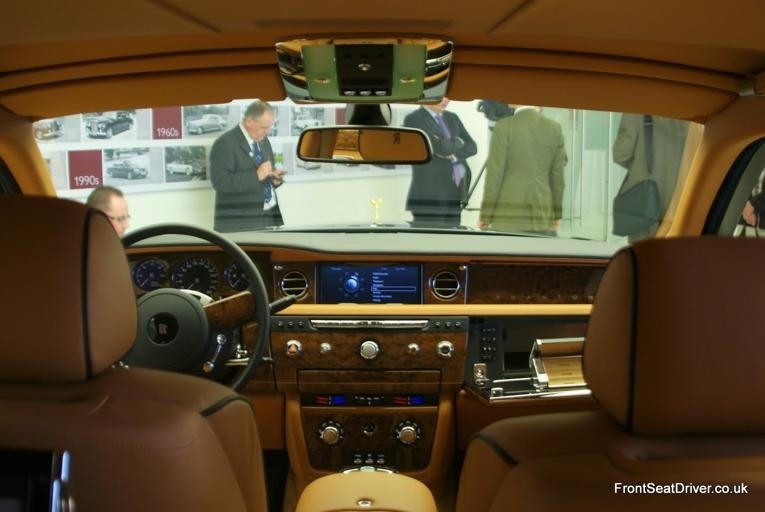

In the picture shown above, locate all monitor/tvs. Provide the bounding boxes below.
[0,448,72,512]
[315,262,422,304]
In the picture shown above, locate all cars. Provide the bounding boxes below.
[165,158,205,175]
[295,155,321,169]
[32,117,64,140]
[293,114,320,129]
[106,160,148,180]
[187,114,228,134]
[84,110,134,139]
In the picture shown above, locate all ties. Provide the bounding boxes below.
[436,114,461,187]
[253,142,272,203]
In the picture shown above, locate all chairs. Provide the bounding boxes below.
[0,193,764,512]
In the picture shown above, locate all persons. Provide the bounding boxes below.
[612,111,691,246]
[87,186,131,237]
[477,102,569,238]
[210,100,288,233]
[404,96,478,227]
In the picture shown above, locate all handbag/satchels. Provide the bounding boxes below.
[612,180,662,235]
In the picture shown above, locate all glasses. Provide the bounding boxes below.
[108,215,130,222]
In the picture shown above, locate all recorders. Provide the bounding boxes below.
[300,392,438,471]
